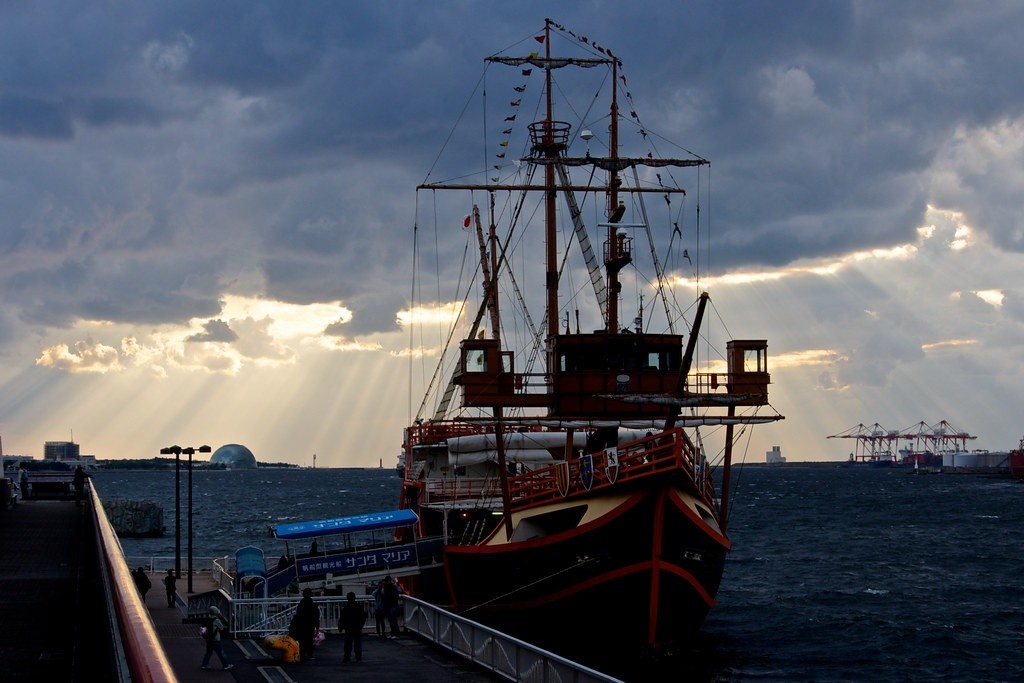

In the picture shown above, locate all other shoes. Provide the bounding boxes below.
[168,605,175,608]
[342,659,351,662]
[378,632,385,635]
[357,660,362,664]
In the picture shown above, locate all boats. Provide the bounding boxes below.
[2,429,98,476]
[1008,439,1024,478]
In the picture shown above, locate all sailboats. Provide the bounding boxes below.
[255,17,785,665]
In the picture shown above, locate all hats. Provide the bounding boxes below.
[209,606,220,618]
[348,593,357,601]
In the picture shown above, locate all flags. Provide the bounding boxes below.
[647,152,689,259]
[490,35,546,182]
[463,212,476,233]
[548,20,623,68]
[478,354,483,365]
[620,74,647,139]
[478,329,484,340]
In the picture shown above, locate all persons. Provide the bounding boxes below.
[309,542,317,556]
[164,569,176,609]
[74,464,94,499]
[337,591,367,664]
[201,606,235,670]
[646,430,658,462]
[372,576,401,639]
[286,587,320,660]
[18,462,29,499]
[135,567,151,603]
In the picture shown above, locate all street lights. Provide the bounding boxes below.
[181,445,211,594]
[160,445,182,578]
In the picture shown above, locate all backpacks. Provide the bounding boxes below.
[199,615,218,643]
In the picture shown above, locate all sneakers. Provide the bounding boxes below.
[388,635,401,640]
[224,663,234,670]
[202,664,211,670]
